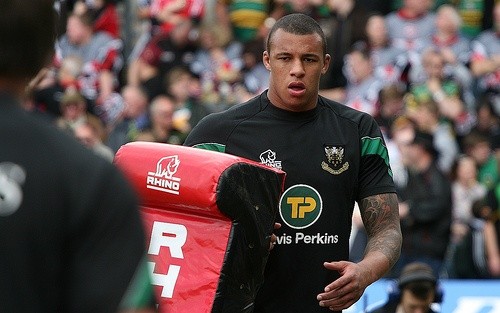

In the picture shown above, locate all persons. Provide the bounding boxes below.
[182,12,404,313]
[26,0,500,313]
[0,0,158,313]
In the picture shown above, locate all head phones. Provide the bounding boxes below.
[388,272,443,304]
